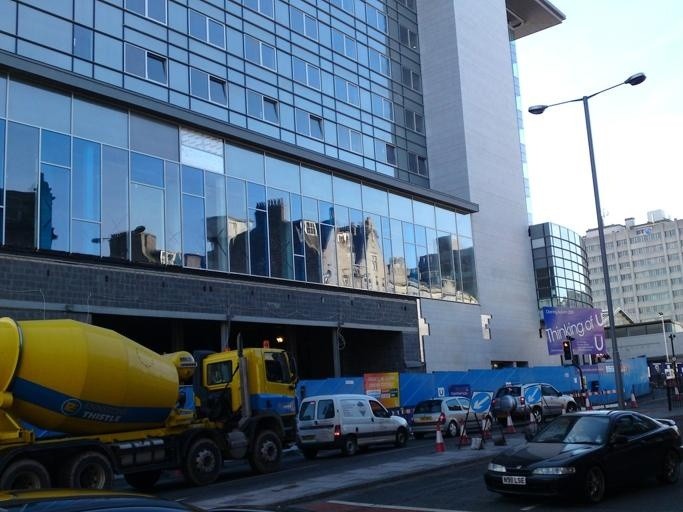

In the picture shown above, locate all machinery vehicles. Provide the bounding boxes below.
[0,316,299,493]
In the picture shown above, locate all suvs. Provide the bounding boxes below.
[295,393,414,458]
[491,383,577,425]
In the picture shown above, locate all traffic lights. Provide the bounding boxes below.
[563,341,571,360]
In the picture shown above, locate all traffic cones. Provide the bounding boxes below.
[674,386,681,401]
[435,409,539,453]
[585,392,593,411]
[630,392,638,409]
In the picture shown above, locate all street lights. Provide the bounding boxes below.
[528,72,646,412]
[657,312,670,369]
[92,226,145,244]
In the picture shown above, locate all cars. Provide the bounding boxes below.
[409,396,492,439]
[484,410,682,503]
